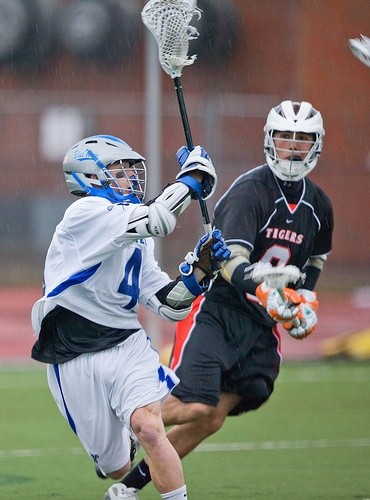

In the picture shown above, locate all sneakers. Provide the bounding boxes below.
[96,436,137,480]
[105,483,138,500]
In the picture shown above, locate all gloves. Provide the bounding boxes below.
[283,289,318,340]
[178,229,232,297]
[176,145,217,200]
[256,281,301,324]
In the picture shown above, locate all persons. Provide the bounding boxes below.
[31,135,230,500]
[105,101,335,500]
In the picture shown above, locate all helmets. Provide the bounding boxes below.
[62,134,147,204]
[262,100,326,183]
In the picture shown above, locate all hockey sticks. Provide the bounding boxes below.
[141,0,225,271]
[243,261,308,329]
